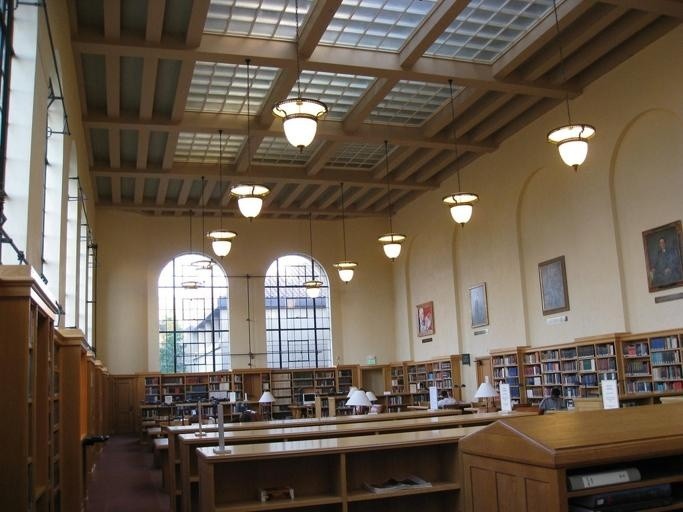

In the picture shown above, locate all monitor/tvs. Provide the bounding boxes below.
[303,393,316,404]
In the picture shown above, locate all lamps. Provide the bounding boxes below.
[365,392,378,402]
[345,390,373,414]
[473,381,498,412]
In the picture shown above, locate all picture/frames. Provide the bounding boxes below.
[537,255,570,316]
[642,219,683,293]
[469,281,489,329]
[416,301,435,337]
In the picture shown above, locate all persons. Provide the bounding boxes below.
[648,233,681,281]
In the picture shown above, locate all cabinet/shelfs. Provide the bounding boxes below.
[389,328,682,411]
[139,365,358,446]
[0,280,114,511]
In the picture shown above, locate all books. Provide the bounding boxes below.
[256,484,297,502]
[565,466,646,491]
[365,473,434,494]
[389,335,683,414]
[262,369,353,418]
[144,375,244,421]
